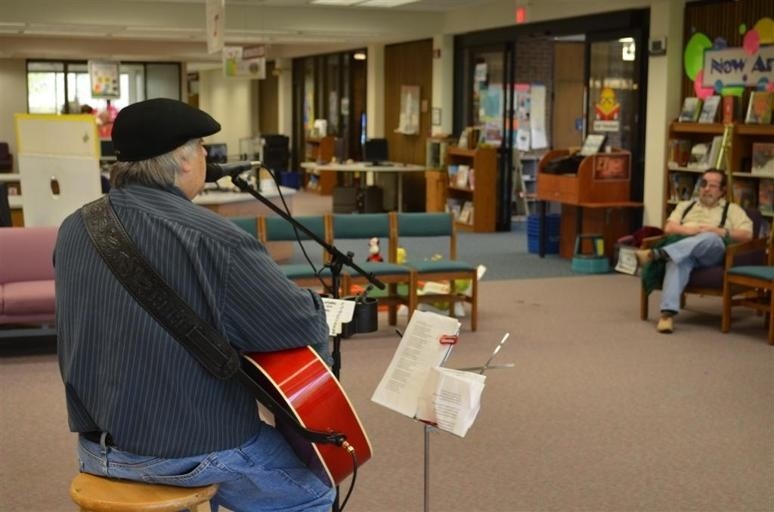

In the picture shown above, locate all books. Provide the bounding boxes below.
[758,223,773,243]
[667,89,773,217]
[443,125,480,227]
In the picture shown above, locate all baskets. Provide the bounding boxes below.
[527,215,560,254]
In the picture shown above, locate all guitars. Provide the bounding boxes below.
[240,344,375,490]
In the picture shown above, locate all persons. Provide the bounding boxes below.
[398,89,420,135]
[50,98,339,512]
[95,110,113,138]
[632,167,754,334]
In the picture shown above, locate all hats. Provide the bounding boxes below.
[111,97,222,160]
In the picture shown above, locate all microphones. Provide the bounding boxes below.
[206,160,263,182]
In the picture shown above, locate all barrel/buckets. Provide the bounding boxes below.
[528,200,546,214]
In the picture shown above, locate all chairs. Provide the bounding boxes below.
[224,210,478,333]
[1,226,68,338]
[638,213,774,346]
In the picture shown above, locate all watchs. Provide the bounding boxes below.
[721,227,730,239]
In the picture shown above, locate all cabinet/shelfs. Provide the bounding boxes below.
[444,141,497,233]
[662,113,773,226]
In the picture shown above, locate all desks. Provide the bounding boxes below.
[301,159,426,217]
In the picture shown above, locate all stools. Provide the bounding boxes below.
[68,474,218,512]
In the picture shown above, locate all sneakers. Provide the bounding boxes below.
[635,249,653,266]
[657,316,673,333]
